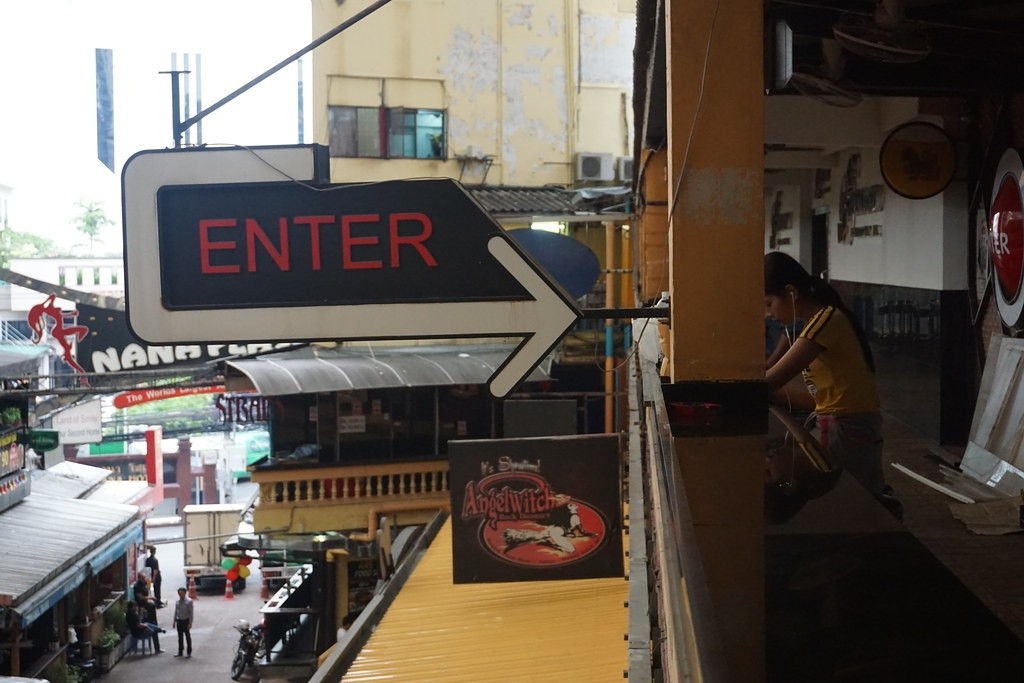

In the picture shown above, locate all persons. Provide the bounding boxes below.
[337,615,354,640]
[763,251,903,517]
[134,572,157,625]
[173,587,193,658]
[145,547,164,608]
[126,601,167,654]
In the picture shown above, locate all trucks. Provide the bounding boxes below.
[181,503,245,592]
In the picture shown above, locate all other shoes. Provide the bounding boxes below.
[186,655,191,658]
[157,649,163,652]
[162,629,166,633]
[175,654,182,657]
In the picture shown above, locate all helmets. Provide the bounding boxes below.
[238,619,250,633]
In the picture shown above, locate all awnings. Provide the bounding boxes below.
[10,521,144,630]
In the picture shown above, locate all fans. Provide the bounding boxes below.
[790,40,863,109]
[832,0,932,63]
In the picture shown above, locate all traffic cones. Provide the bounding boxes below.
[224,577,236,599]
[189,575,199,598]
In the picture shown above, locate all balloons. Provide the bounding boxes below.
[221,555,252,582]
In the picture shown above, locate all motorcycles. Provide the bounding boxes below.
[231,624,268,680]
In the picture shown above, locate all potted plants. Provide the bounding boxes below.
[97,598,136,671]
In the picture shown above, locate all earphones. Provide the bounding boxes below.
[790,292,795,303]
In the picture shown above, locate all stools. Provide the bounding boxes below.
[132,634,152,656]
